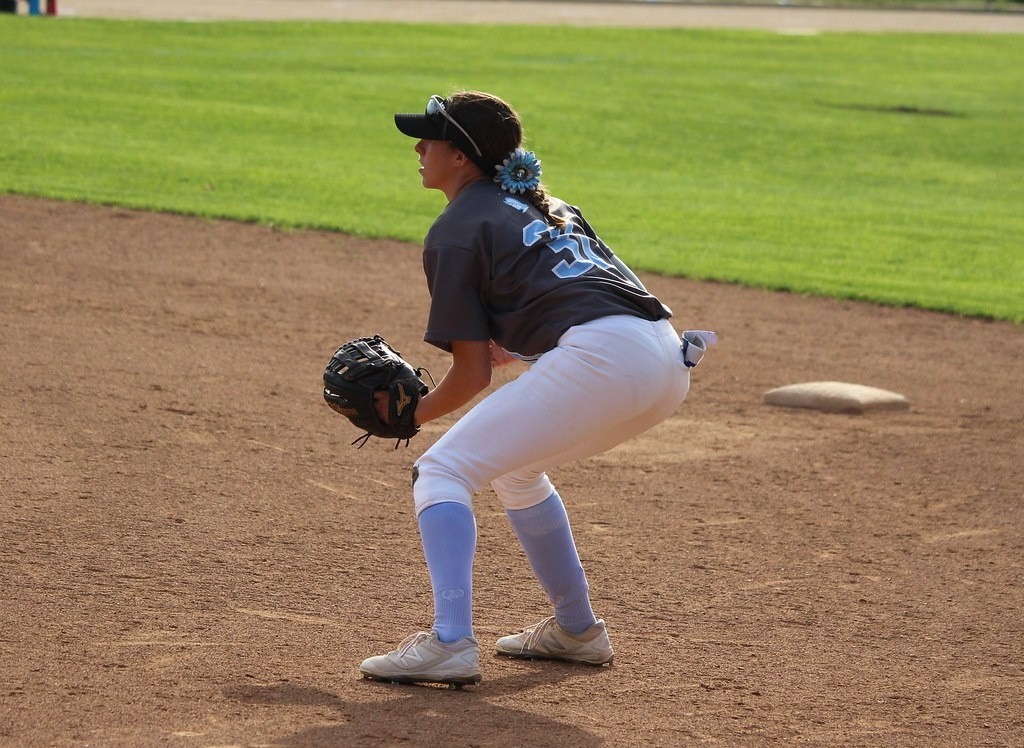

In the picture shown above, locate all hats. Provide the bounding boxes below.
[394,113,490,166]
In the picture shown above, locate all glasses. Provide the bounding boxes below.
[424,94,483,158]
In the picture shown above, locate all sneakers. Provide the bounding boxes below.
[495,617,614,667]
[359,630,483,687]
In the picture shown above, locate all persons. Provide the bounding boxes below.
[322,90,691,681]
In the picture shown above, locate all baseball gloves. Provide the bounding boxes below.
[322,330,431,441]
